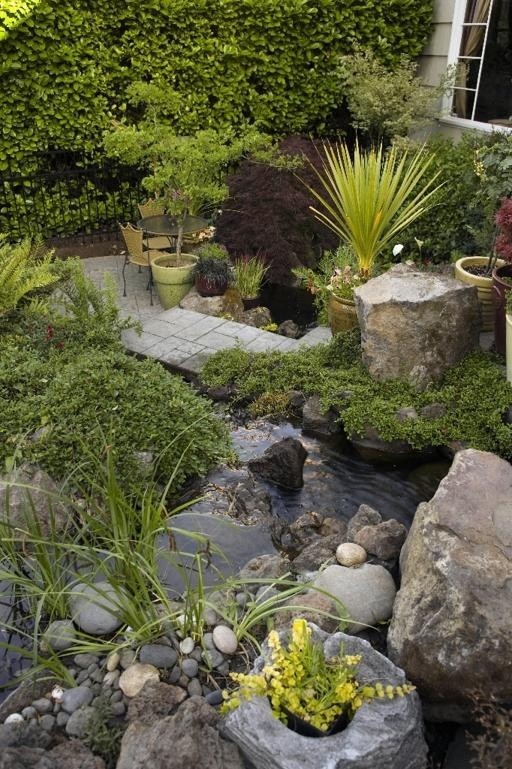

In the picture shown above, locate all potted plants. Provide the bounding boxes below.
[454,196,512,384]
[100,118,307,313]
[290,131,454,345]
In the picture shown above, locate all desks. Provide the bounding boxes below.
[136,213,209,253]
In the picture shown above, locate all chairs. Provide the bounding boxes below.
[116,196,182,308]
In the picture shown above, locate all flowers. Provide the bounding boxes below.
[216,615,418,732]
